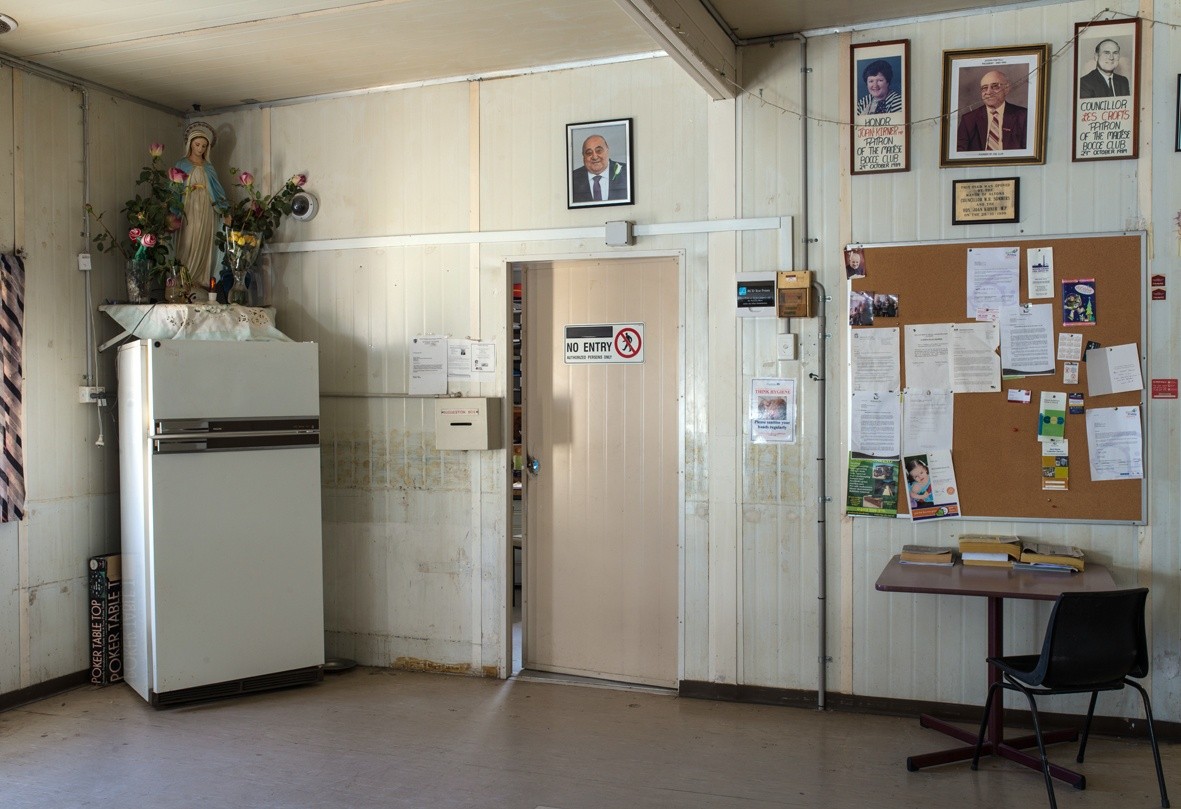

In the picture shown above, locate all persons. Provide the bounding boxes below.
[156,122,230,287]
[857,61,902,115]
[851,295,896,325]
[1080,39,1130,98]
[573,135,627,203]
[906,459,934,506]
[846,253,864,278]
[956,70,1028,151]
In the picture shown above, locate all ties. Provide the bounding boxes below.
[1108,78,1113,96]
[592,176,602,201]
[986,111,1001,151]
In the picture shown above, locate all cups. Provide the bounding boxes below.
[165,265,192,304]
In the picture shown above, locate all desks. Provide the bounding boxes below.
[875,553,1121,794]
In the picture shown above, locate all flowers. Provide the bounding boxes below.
[76,143,307,307]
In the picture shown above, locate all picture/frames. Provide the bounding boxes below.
[939,42,1049,168]
[850,39,910,175]
[566,118,635,209]
[1072,17,1142,163]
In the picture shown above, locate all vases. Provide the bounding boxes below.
[123,258,157,304]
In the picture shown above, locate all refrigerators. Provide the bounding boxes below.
[114,338,326,710]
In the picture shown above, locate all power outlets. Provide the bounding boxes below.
[78,386,105,404]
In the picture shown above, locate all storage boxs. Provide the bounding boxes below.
[88,553,124,688]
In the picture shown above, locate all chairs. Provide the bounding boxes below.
[971,587,1171,809]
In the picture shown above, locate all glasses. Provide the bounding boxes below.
[981,83,1008,92]
